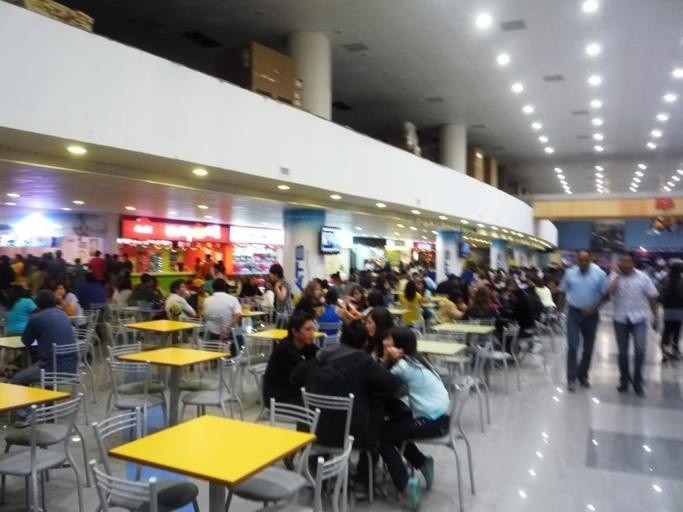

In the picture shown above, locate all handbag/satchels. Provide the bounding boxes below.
[276,283,291,330]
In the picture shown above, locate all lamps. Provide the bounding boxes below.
[10,213,64,238]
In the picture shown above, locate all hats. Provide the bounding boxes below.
[192,279,206,289]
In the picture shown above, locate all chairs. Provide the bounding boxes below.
[180,345,247,422]
[302,387,388,510]
[380,373,477,512]
[4,368,87,512]
[225,397,321,510]
[71,326,97,403]
[478,321,522,393]
[79,308,102,361]
[105,357,163,439]
[447,343,492,435]
[230,325,259,395]
[88,459,159,511]
[105,341,166,415]
[0,391,85,512]
[178,338,232,420]
[50,339,88,402]
[251,434,356,512]
[106,322,128,347]
[91,405,201,512]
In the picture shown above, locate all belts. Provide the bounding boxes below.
[569,305,583,312]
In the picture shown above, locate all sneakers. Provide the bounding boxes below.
[398,476,422,511]
[567,379,575,393]
[578,378,592,389]
[633,384,644,397]
[422,456,434,491]
[15,418,32,428]
[352,483,380,502]
[616,385,629,392]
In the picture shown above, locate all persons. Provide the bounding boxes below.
[13,290,78,422]
[366,289,392,326]
[400,280,429,330]
[129,274,155,305]
[378,328,452,512]
[240,275,262,299]
[429,263,557,360]
[346,285,367,313]
[659,258,683,363]
[1,250,133,306]
[262,310,319,468]
[187,279,212,318]
[353,261,429,289]
[2,285,38,336]
[314,289,350,337]
[269,264,293,329]
[165,279,197,322]
[557,250,610,393]
[315,319,403,496]
[202,278,243,358]
[289,281,324,329]
[196,254,228,294]
[606,255,660,398]
[56,282,88,339]
[364,306,395,363]
